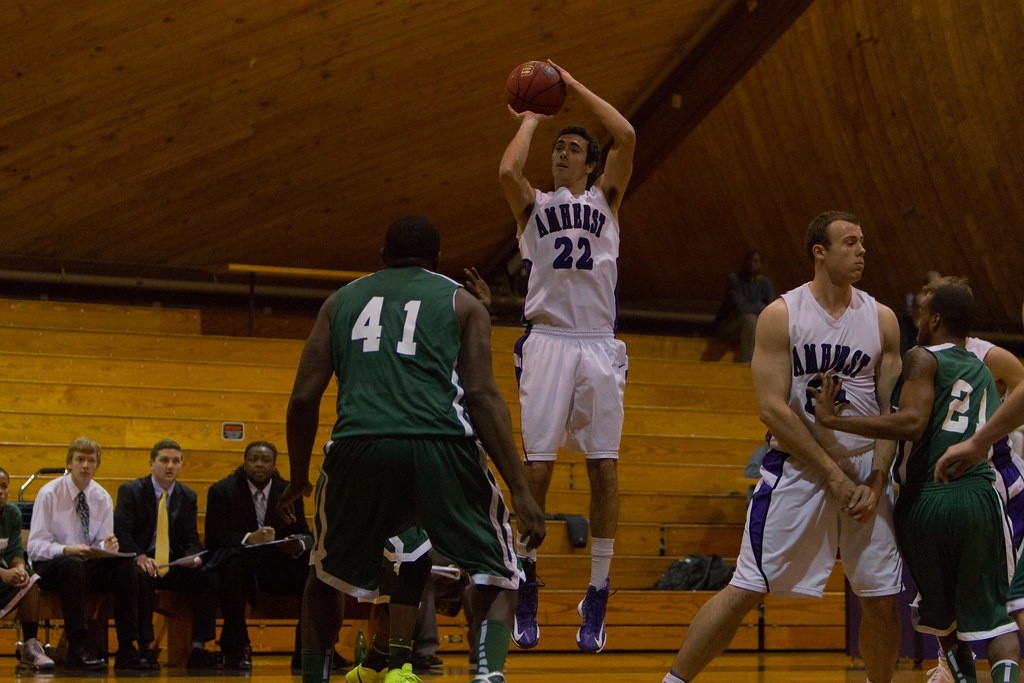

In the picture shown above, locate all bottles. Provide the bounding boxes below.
[439,634,466,645]
[355,628,368,666]
[257,624,268,647]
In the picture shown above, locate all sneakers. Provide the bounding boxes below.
[509,582,541,649]
[345,663,389,683]
[413,654,444,670]
[384,663,425,682]
[576,578,611,653]
[21,638,55,668]
[927,657,955,683]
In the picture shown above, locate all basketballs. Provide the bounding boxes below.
[504,60,567,117]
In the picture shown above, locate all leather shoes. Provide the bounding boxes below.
[138,648,159,668]
[114,647,149,669]
[64,647,104,671]
[225,659,252,668]
[331,652,353,668]
[186,647,223,669]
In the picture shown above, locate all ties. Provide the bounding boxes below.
[76,492,90,546]
[155,489,169,577]
[252,491,267,529]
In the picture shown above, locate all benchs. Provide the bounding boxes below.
[0,299,847,652]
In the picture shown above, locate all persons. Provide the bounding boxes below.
[661,210,906,683]
[345,267,491,683]
[498,59,636,653]
[808,276,1024,683]
[410,547,478,668]
[0,467,54,669]
[204,440,315,669]
[712,250,776,363]
[933,381,1024,485]
[113,438,223,670]
[919,277,1024,683]
[277,215,547,683]
[27,437,150,670]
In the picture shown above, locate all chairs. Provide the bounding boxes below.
[1,500,56,651]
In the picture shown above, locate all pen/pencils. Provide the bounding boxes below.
[257,518,263,527]
[104,529,110,535]
